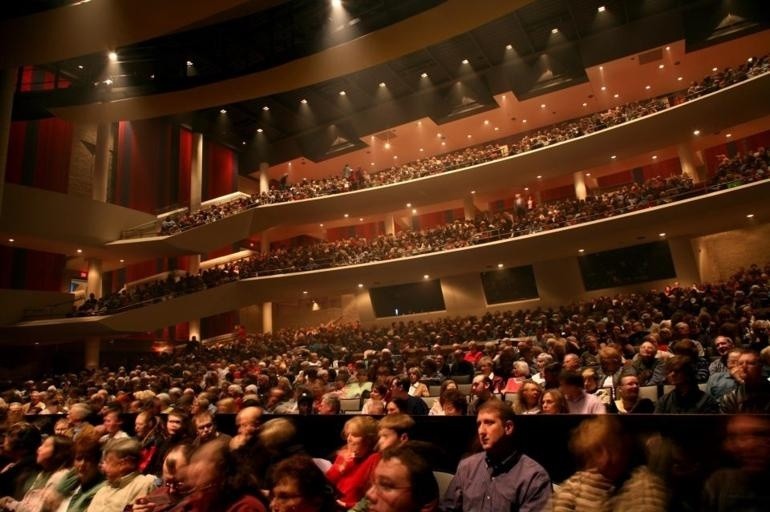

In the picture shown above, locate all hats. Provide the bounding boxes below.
[297,392,313,404]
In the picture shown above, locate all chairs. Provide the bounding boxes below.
[339,337,770,512]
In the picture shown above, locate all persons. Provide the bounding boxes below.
[158,144,769,292]
[1,257,769,512]
[509,51,769,156]
